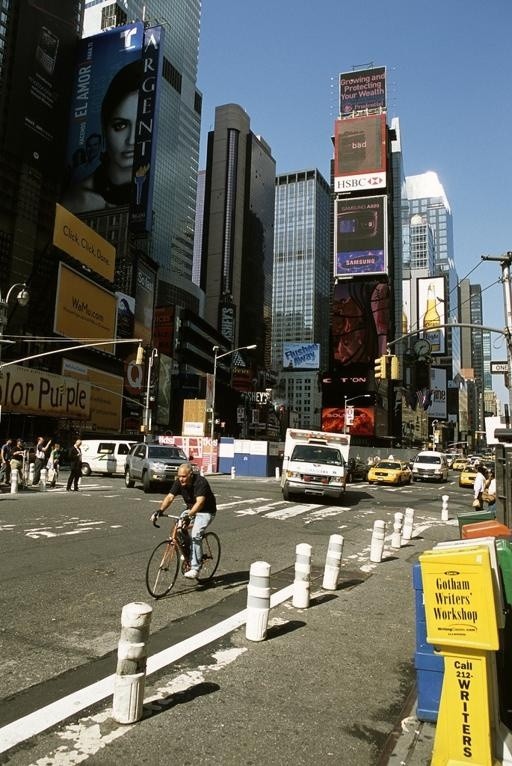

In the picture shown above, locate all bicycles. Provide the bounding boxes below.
[146,512,220,599]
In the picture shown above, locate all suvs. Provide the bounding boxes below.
[124,442,200,494]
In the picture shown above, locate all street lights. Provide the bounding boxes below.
[432,419,445,450]
[207,344,259,473]
[145,347,158,444]
[344,394,371,434]
[409,419,426,448]
[0,281,29,424]
[244,388,273,439]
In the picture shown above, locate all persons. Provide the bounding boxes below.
[152,463,213,578]
[0,436,84,493]
[484,469,495,512]
[474,466,486,510]
[63,58,146,213]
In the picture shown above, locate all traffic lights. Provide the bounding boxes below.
[374,356,384,379]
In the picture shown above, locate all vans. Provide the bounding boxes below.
[78,439,142,476]
[410,451,449,484]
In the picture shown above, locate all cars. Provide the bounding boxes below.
[21,441,38,460]
[367,455,412,487]
[409,450,495,478]
[59,446,77,468]
[458,459,491,487]
[346,457,370,483]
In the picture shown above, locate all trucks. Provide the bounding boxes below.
[278,427,355,506]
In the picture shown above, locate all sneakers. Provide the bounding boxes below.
[182,568,200,579]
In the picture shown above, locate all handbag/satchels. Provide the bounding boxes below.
[481,482,497,504]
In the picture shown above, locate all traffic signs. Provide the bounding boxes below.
[491,360,508,373]
